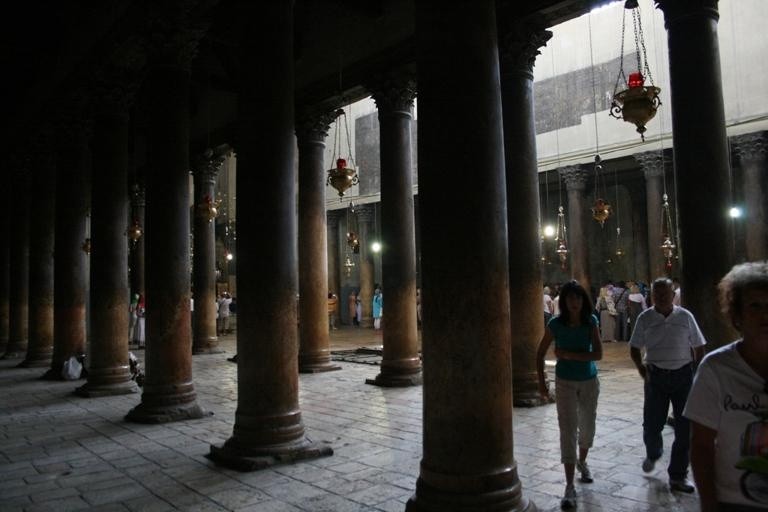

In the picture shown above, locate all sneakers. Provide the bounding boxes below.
[576,459,593,482]
[643,454,661,471]
[561,486,576,507]
[669,478,695,492]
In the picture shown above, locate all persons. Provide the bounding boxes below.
[536,278,603,511]
[627,277,708,493]
[683,260,767,511]
[542,277,683,343]
[126,276,421,351]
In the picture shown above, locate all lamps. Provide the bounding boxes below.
[554,0,678,271]
[80,216,91,254]
[128,206,144,246]
[325,107,360,250]
[197,190,222,223]
